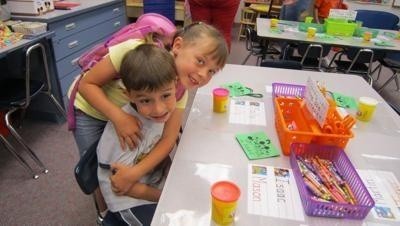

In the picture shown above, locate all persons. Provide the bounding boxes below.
[67,13,228,226]
[315,0,347,68]
[95,44,177,226]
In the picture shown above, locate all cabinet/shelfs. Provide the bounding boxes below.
[11,0,128,115]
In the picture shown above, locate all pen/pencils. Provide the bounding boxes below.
[296,155,359,216]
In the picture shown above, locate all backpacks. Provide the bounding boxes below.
[67,11,188,130]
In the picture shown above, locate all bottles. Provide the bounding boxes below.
[269,19,279,32]
[355,97,378,122]
[212,88,229,113]
[307,27,317,39]
[364,31,372,42]
[356,20,363,28]
[209,180,242,226]
[304,16,314,24]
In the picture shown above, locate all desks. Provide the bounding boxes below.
[0,31,55,57]
[249,4,282,18]
[256,18,400,50]
[126,0,192,27]
[149,64,400,226]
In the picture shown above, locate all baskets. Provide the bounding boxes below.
[324,17,356,36]
[274,96,354,161]
[289,142,375,220]
[271,81,335,101]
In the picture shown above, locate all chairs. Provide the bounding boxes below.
[143,1,175,25]
[346,11,399,63]
[334,47,383,86]
[242,27,280,66]
[283,42,331,70]
[237,7,256,42]
[0,37,70,179]
[261,60,302,70]
[369,52,400,94]
[73,138,157,225]
